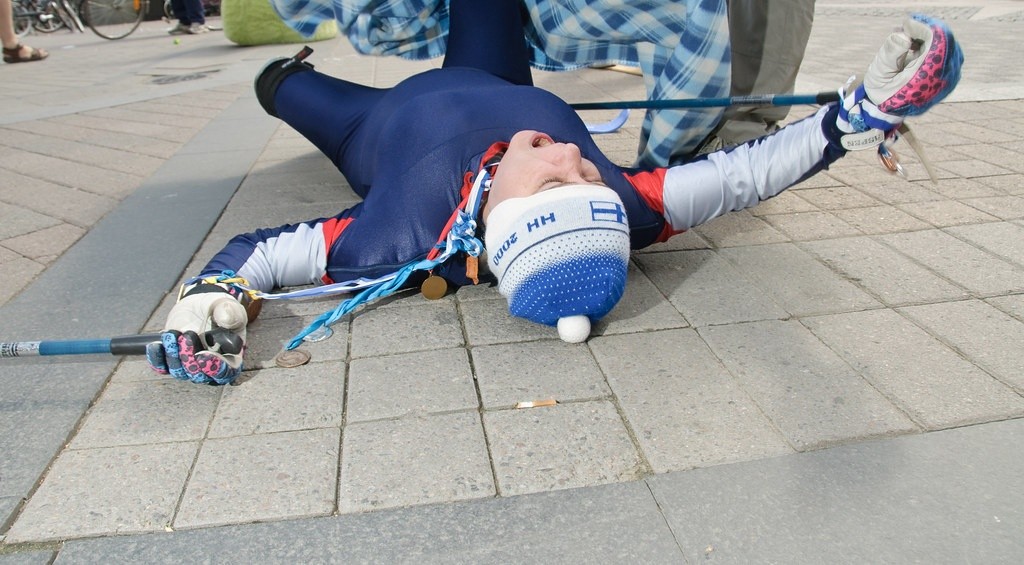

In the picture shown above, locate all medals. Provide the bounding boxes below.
[276,349,311,368]
[302,326,333,341]
[421,276,447,300]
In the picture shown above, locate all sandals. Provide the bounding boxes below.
[3,42,49,63]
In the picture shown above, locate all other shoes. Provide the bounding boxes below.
[187,22,211,34]
[685,133,740,165]
[168,22,191,36]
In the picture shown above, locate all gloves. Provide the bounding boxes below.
[836,15,964,151]
[146,270,251,385]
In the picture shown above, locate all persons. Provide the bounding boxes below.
[166,0,205,37]
[0,0,49,63]
[146,0,964,391]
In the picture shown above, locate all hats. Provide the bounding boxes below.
[484,184,631,343]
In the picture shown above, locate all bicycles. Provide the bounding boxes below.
[11,1,224,40]
